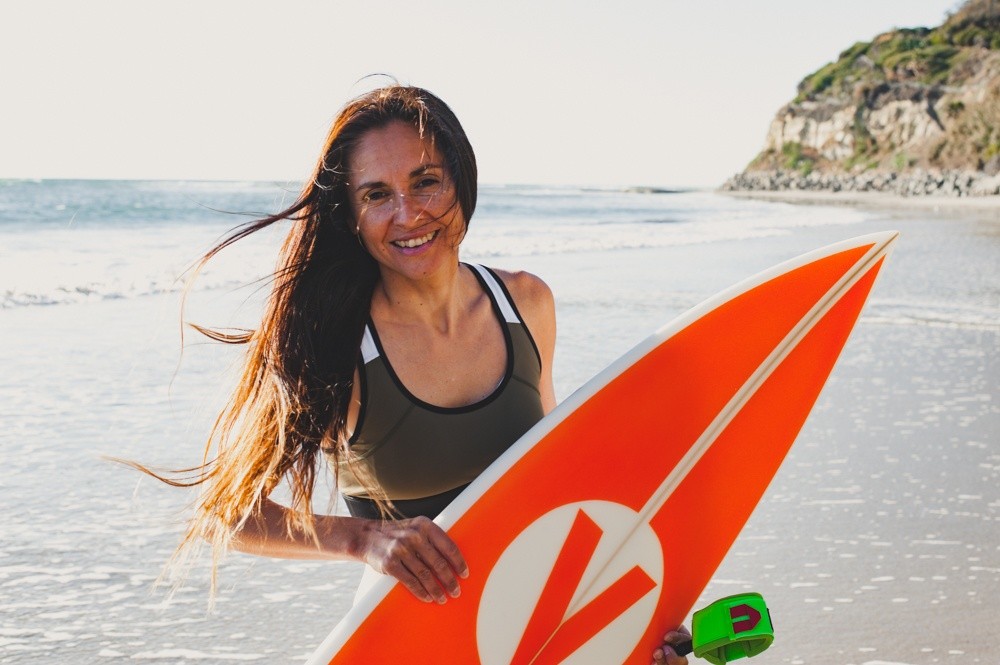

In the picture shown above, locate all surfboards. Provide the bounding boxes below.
[303,230,903,665]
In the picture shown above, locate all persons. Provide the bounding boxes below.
[101,86,692,665]
